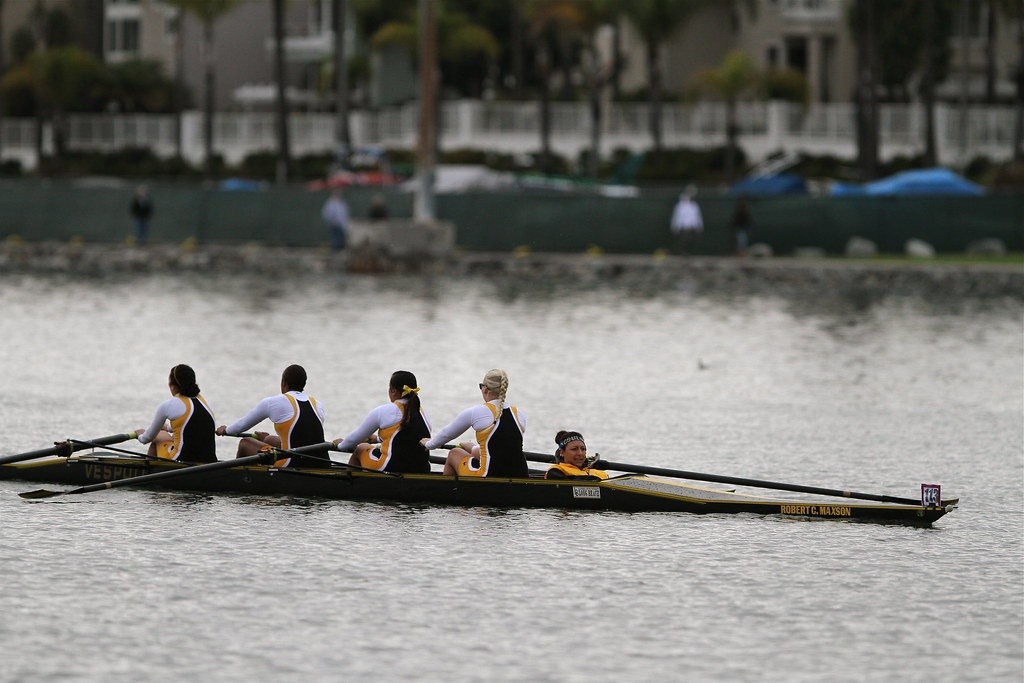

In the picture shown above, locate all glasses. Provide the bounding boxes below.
[479,383,491,390]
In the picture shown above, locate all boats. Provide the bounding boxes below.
[0,451,959,527]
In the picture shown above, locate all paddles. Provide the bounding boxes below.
[0,433,960,505]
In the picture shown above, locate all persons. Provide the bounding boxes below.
[545,430,609,480]
[325,189,348,250]
[130,183,154,247]
[333,370,432,474]
[672,193,701,253]
[372,191,389,218]
[135,364,217,463]
[420,369,529,478]
[216,364,331,468]
